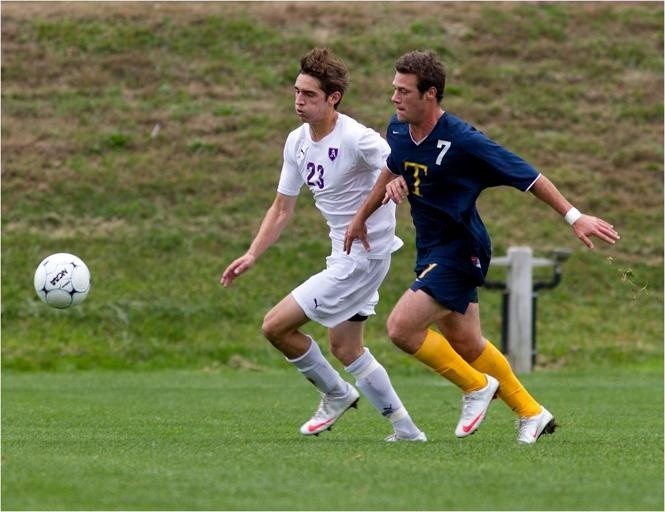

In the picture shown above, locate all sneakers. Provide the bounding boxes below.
[513,404,555,444]
[454,372,501,438]
[384,431,427,443]
[300,382,361,437]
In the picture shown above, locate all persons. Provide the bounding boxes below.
[341,50,622,444]
[221,45,430,445]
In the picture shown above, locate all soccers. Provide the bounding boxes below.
[33,252,90,308]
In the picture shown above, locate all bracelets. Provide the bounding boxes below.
[563,206,583,226]
